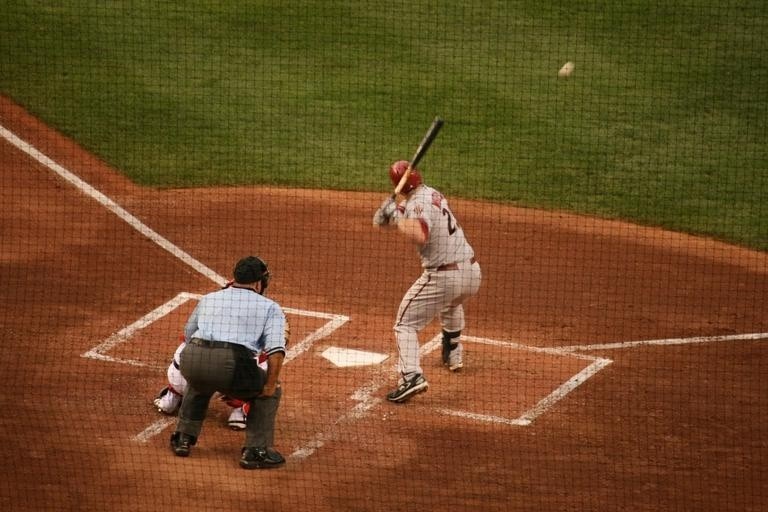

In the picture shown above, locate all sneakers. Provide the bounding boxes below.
[153,398,174,414]
[171,432,190,457]
[446,342,463,371]
[228,412,248,430]
[387,373,429,402]
[240,448,286,470]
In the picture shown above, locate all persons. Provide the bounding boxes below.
[152,338,269,431]
[169,256,286,470]
[372,161,482,402]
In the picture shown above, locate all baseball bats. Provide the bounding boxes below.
[390,114,442,199]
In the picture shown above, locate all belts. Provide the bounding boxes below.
[438,257,476,271]
[190,337,255,355]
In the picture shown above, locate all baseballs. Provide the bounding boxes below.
[559,62,573,77]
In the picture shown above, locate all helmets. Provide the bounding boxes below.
[388,161,421,194]
[233,255,269,295]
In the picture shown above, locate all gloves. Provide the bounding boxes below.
[375,199,396,226]
